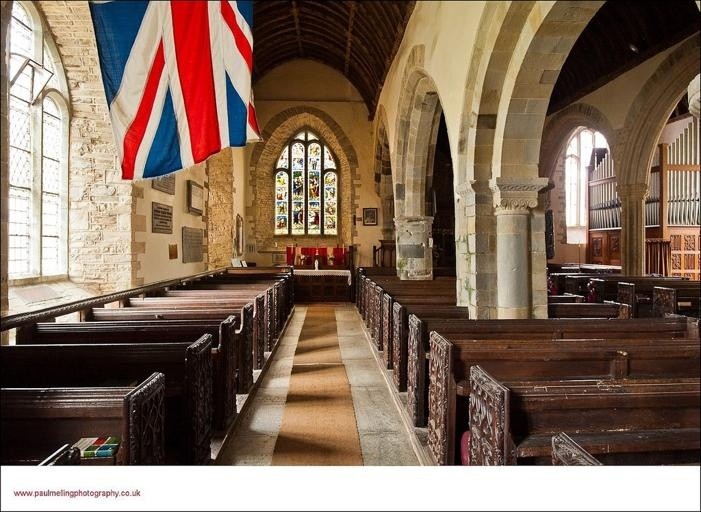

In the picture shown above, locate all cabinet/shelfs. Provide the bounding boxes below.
[293,269,352,303]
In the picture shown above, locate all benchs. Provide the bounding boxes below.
[0,264,296,465]
[353,262,701,466]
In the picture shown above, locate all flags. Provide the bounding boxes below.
[86,0,264,181]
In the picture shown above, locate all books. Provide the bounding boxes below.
[71,436,121,458]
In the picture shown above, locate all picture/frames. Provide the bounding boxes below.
[236,213,244,256]
[362,207,378,225]
